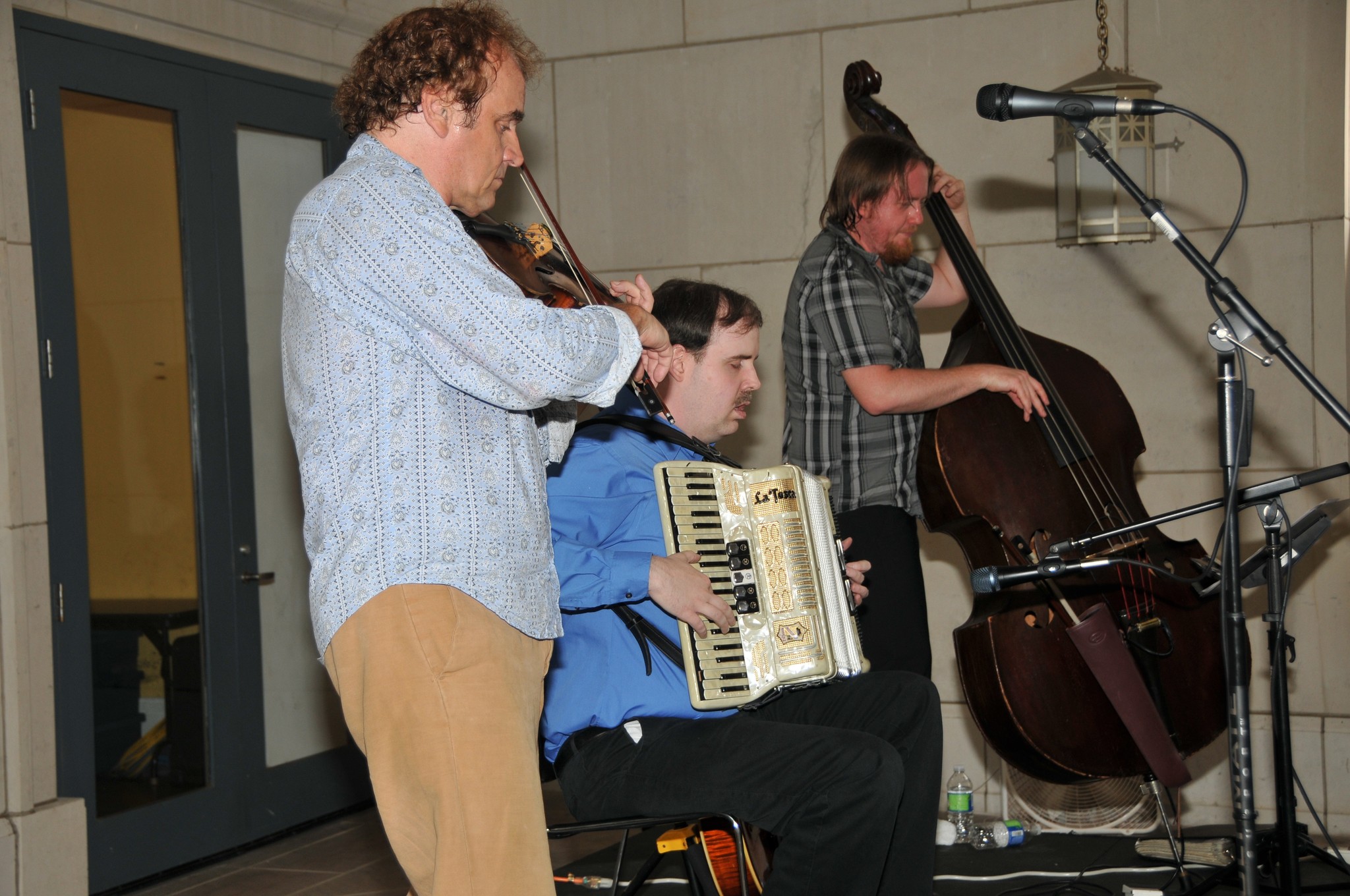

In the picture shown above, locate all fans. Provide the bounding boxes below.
[1001,758,1162,834]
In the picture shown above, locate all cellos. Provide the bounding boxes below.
[841,51,1254,895]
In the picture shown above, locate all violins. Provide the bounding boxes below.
[456,208,623,309]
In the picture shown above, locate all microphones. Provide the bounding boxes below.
[976,83,1174,122]
[971,555,1124,593]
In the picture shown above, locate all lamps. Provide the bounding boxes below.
[1047,0,1185,248]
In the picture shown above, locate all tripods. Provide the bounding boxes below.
[1049,462,1350,896]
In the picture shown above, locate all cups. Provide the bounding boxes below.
[936,820,957,845]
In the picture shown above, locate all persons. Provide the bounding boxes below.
[781,133,1050,682]
[281,1,672,896]
[538,279,943,896]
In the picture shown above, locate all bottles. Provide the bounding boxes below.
[946,767,975,844]
[967,818,1042,849]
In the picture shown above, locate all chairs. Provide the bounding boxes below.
[537,732,749,896]
